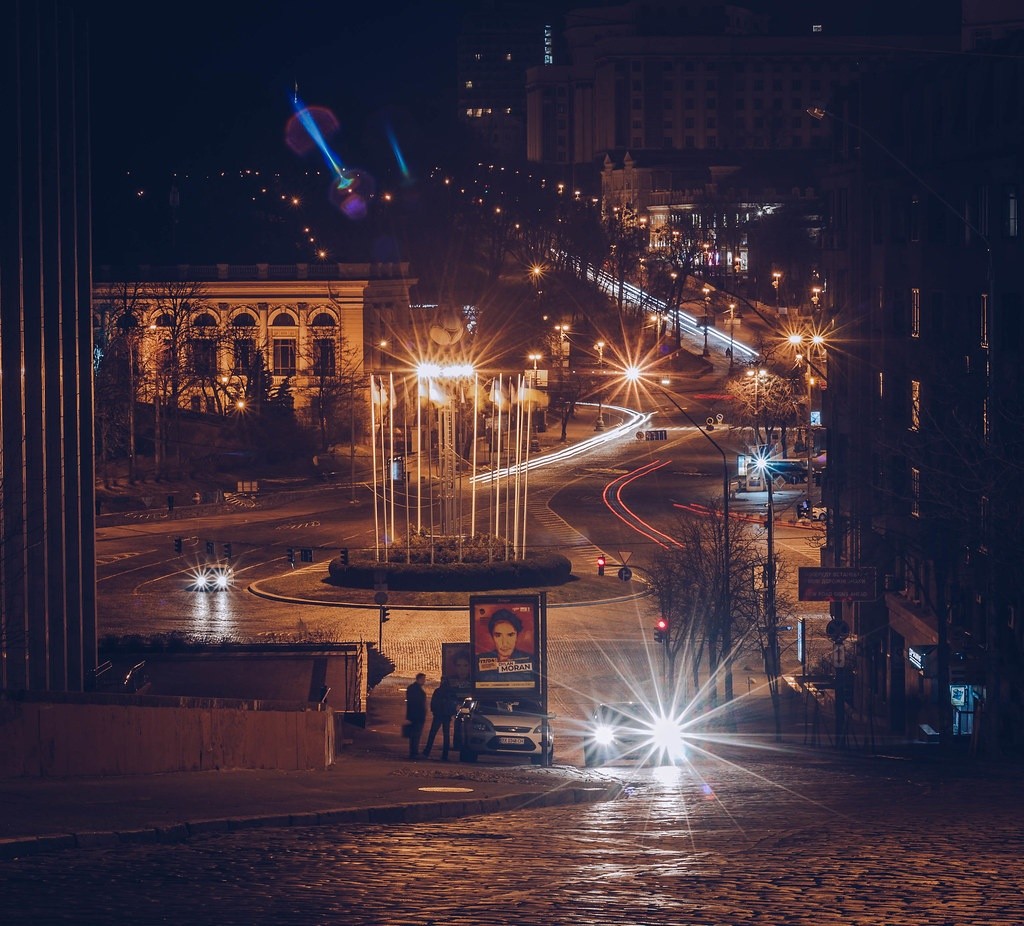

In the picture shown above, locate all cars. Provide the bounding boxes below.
[810,500,827,522]
[583,701,684,767]
[453,696,556,766]
[194,564,235,590]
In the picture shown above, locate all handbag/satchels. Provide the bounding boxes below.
[402,724,411,738]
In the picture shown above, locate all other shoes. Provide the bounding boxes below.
[409,756,421,761]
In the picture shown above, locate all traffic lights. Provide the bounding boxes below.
[341,550,349,565]
[223,543,232,558]
[287,548,296,563]
[597,556,606,576]
[206,541,214,554]
[174,538,182,553]
[653,621,666,643]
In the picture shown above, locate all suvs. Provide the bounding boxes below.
[764,458,815,482]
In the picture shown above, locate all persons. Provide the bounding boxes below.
[423,676,457,761]
[452,650,470,679]
[406,673,426,760]
[477,609,532,663]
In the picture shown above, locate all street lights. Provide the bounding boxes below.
[789,334,825,505]
[555,323,570,395]
[773,272,782,319]
[634,257,646,322]
[670,271,677,337]
[812,287,822,324]
[702,287,711,357]
[746,366,767,445]
[596,339,606,431]
[726,303,737,375]
[529,353,543,453]
